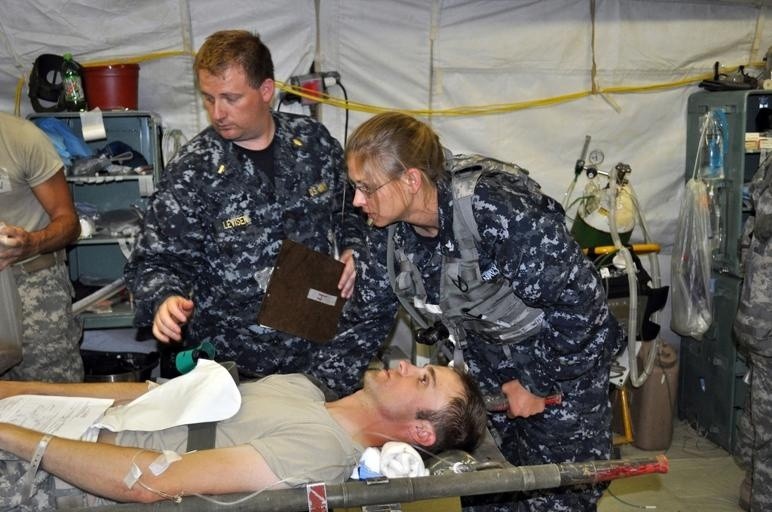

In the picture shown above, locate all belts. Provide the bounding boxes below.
[15,253,56,274]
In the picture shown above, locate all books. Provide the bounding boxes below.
[258,237,349,346]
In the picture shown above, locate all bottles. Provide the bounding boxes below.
[60,53,85,112]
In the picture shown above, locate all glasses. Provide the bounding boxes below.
[349,180,392,199]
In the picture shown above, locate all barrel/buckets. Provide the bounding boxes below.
[82,62,143,113]
[568,200,633,258]
[611,344,681,454]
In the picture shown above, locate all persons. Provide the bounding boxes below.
[126,31,369,378]
[303,111,625,511]
[1,113,88,383]
[731,152,772,509]
[1,360,488,512]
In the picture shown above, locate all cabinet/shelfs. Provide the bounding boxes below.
[24,109,161,383]
